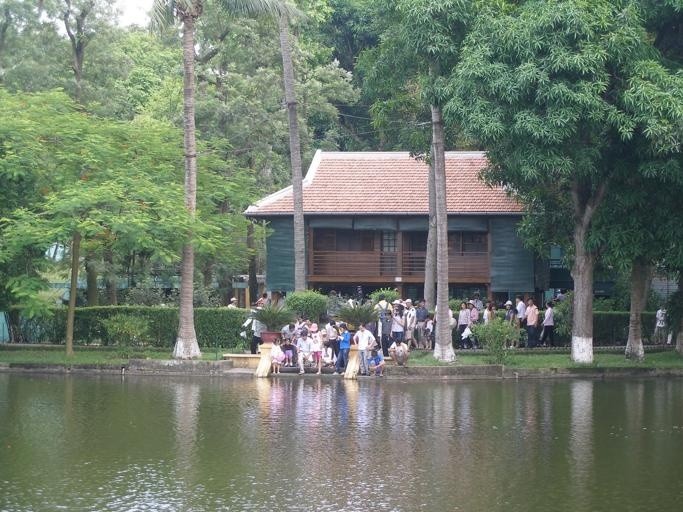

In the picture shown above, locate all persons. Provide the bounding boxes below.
[227,295,238,308]
[652,303,668,345]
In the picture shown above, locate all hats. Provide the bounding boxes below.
[392,298,415,313]
[231,298,239,302]
[310,320,348,331]
[467,300,513,307]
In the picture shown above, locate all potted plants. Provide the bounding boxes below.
[247,305,296,343]
[334,302,379,345]
